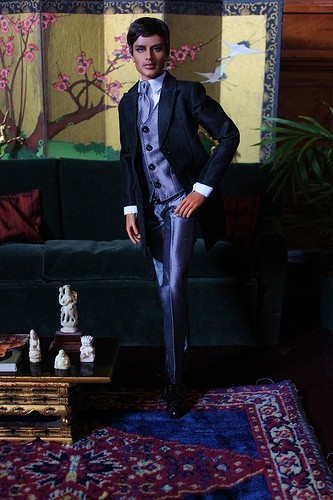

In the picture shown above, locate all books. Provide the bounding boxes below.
[0,334,30,372]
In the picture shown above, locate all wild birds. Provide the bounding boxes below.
[211,29,270,65]
[192,57,239,91]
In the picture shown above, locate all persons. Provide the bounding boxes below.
[117,17,241,417]
[30,285,94,370]
[0,334,28,361]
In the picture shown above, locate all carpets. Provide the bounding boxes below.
[0,378,333,500]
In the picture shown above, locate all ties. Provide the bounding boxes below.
[138,81,151,124]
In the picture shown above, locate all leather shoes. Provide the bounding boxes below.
[170,391,192,417]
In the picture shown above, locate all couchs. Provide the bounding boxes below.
[0,158,283,347]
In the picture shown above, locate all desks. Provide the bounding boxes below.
[288,247,333,333]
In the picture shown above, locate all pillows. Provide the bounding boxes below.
[219,192,257,233]
[0,188,44,244]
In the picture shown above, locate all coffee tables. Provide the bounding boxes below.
[0,337,122,444]
